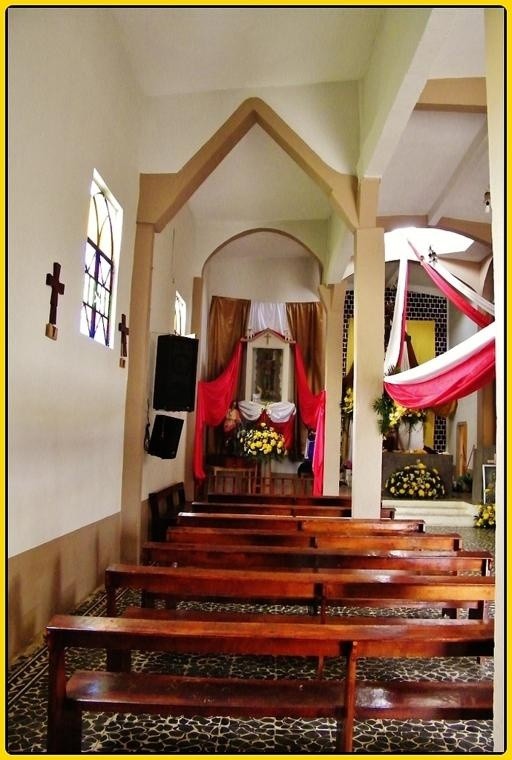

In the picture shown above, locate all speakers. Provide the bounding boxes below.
[152,333,201,413]
[145,414,184,460]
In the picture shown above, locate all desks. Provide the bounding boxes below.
[204,464,256,494]
[302,472,314,494]
[261,473,299,495]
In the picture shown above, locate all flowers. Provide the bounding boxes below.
[234,422,288,465]
[473,502,496,527]
[383,459,446,499]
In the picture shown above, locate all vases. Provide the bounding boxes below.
[259,456,271,478]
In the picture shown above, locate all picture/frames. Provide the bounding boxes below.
[481,464,496,504]
